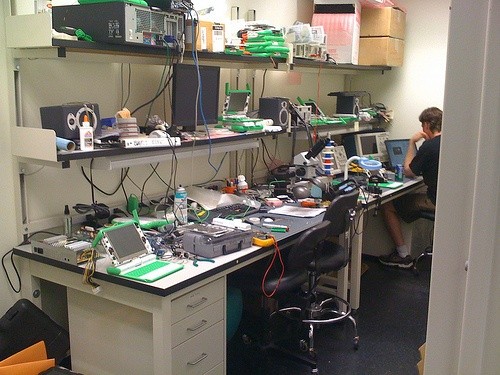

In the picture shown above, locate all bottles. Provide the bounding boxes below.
[79,115,93,151]
[64,204,72,236]
[174,188,187,223]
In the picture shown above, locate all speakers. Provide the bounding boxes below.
[336,96,359,118]
[40,103,100,139]
[259,97,289,128]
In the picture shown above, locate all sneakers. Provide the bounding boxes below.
[378,251,414,270]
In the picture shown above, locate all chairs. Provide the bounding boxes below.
[239,191,359,375]
[412,210,435,274]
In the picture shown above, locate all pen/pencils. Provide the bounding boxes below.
[225,176,238,187]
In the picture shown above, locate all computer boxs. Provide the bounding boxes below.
[51,2,186,50]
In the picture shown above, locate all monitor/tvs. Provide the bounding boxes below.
[172,63,220,134]
[360,135,378,156]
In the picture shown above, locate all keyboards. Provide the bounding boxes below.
[202,203,259,223]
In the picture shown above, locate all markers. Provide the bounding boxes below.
[262,223,289,232]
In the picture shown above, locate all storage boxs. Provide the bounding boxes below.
[181,19,226,52]
[310,0,407,67]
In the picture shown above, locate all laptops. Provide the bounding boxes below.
[91,195,185,283]
[384,139,417,170]
[217,83,262,122]
[296,97,357,124]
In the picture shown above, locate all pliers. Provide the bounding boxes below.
[189,256,215,266]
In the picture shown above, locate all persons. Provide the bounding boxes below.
[379,107,442,268]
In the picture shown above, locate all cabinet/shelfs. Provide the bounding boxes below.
[286,56,393,140]
[322,174,425,313]
[12,176,352,375]
[3,0,286,172]
